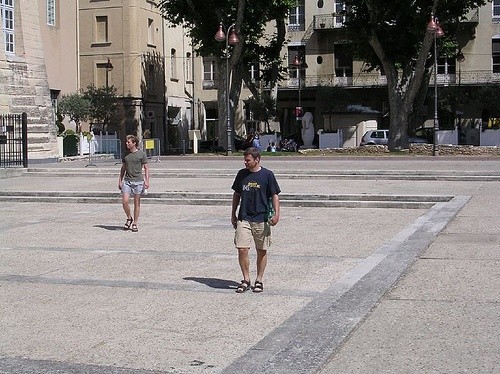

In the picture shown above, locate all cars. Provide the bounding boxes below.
[360,129,430,146]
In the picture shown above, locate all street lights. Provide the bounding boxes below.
[293,54,306,107]
[105,59,114,94]
[427,10,444,156]
[214,21,240,157]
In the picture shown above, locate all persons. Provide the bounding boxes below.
[231,147,281,293]
[118,135,150,232]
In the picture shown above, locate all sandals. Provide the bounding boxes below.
[253,281,264,292]
[131,224,138,232]
[124,217,133,230]
[236,280,252,293]
